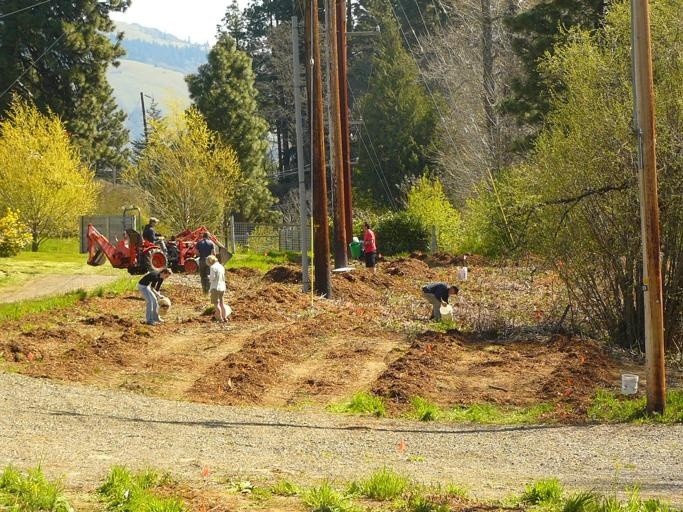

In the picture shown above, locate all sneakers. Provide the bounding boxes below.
[146,317,165,324]
[220,317,229,322]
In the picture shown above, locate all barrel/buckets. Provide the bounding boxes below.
[621,374,638,395]
[440,304,453,319]
[457,267,467,282]
[157,297,171,315]
[350,241,360,258]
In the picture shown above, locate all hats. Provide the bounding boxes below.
[150,217,160,224]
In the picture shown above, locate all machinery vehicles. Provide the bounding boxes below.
[86,206,233,275]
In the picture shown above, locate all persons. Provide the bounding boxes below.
[196,232,228,323]
[422,283,459,319]
[361,222,376,273]
[142,218,169,255]
[137,268,172,325]
